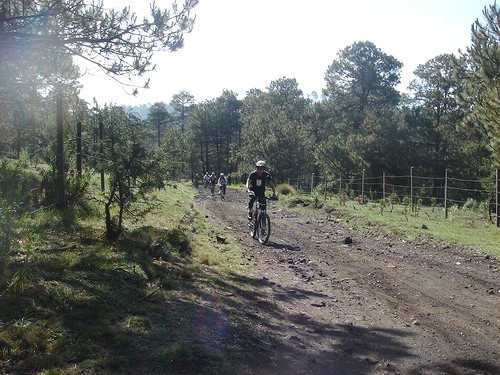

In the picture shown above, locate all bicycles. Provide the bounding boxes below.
[209,182,215,196]
[247,196,276,244]
[194,180,198,188]
[220,186,225,201]
[204,180,208,188]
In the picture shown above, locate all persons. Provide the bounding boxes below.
[194,172,227,195]
[247,161,276,234]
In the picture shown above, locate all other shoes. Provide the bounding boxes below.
[261,228,267,236]
[247,212,253,220]
[218,191,221,195]
[224,196,227,200]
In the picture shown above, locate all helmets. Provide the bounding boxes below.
[256,160,266,167]
[220,173,224,176]
